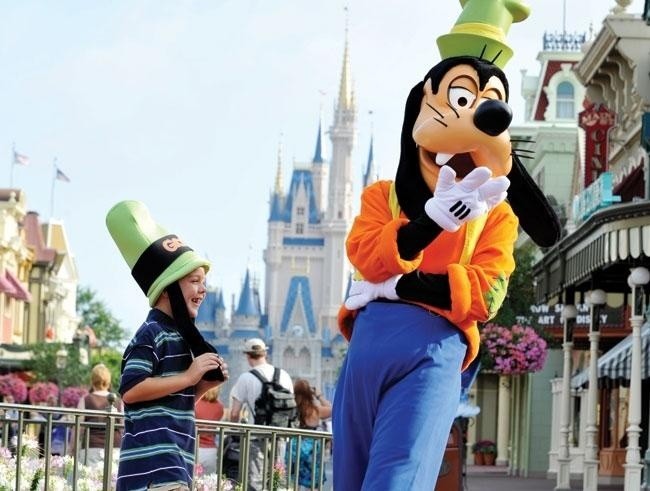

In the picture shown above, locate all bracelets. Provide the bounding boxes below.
[316,394,321,400]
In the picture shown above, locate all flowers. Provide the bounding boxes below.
[479,323,550,379]
[470,441,497,455]
[1,373,86,406]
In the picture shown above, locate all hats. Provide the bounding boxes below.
[243,338,269,354]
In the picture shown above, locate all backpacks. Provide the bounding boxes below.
[246,367,298,429]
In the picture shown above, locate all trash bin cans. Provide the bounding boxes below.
[434,422,464,491]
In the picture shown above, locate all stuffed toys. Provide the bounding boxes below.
[328,1,561,491]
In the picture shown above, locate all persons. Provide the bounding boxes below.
[114,267,231,491]
[285,378,333,491]
[194,385,224,478]
[0,394,73,458]
[68,363,125,468]
[230,337,294,491]
